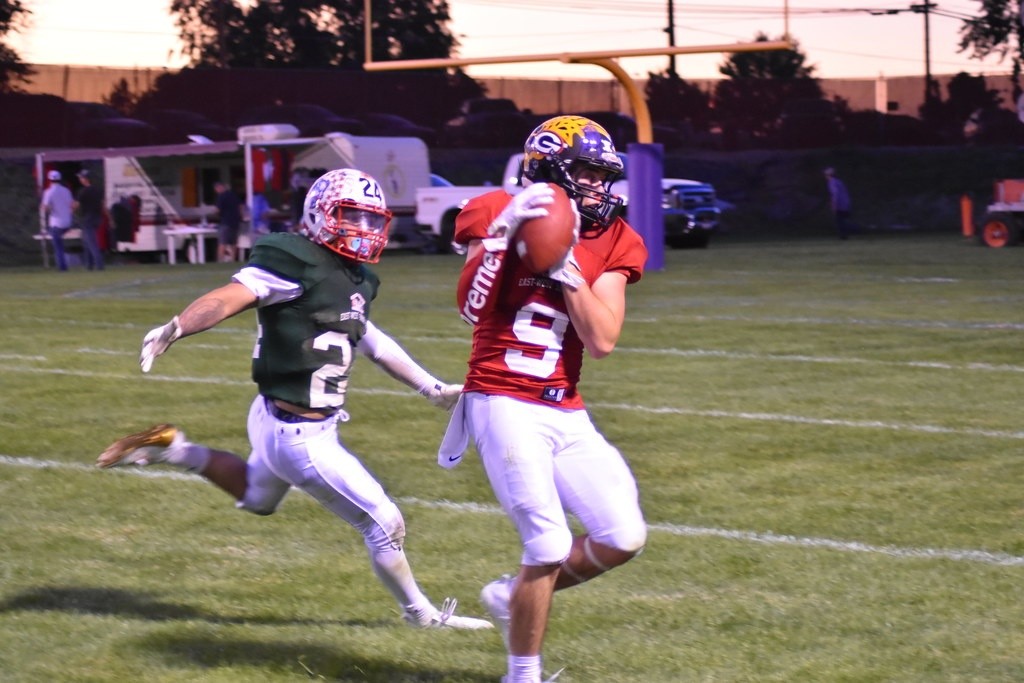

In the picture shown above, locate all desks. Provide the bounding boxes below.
[32,231,82,268]
[162,228,218,264]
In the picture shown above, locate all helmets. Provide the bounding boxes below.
[303,168,393,264]
[521,115,623,227]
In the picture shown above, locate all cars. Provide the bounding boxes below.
[443,98,535,145]
[573,110,639,154]
[343,113,438,148]
[146,109,236,131]
[237,103,363,137]
[61,102,154,128]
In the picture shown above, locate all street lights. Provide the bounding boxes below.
[870,0,937,102]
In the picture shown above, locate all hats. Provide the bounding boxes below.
[76,169,91,178]
[47,170,61,180]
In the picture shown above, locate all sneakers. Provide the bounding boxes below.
[94,423,185,471]
[401,597,494,631]
[481,574,516,651]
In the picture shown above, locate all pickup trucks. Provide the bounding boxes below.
[415,153,724,254]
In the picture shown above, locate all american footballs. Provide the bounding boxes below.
[514,183,576,276]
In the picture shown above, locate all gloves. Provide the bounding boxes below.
[543,199,586,290]
[428,381,464,415]
[482,182,556,252]
[140,316,183,372]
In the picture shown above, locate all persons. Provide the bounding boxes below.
[212,180,240,263]
[40,170,105,272]
[824,168,850,240]
[457,115,648,683]
[252,192,269,233]
[95,169,496,631]
